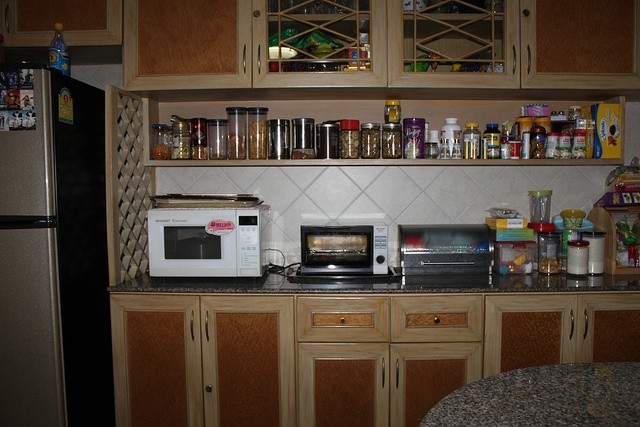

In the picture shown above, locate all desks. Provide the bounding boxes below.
[399,360,638,427]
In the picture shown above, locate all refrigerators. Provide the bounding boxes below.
[0,66,114,426]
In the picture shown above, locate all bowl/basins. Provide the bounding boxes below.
[559,209,586,229]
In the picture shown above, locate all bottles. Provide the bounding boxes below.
[463,122,482,159]
[558,110,566,120]
[190,118,208,159]
[509,140,522,159]
[292,118,315,158]
[47,24,70,76]
[340,120,362,157]
[172,122,191,160]
[523,129,530,160]
[248,107,267,159]
[207,119,230,160]
[516,117,532,138]
[529,191,552,223]
[266,120,292,159]
[586,111,595,159]
[441,118,462,157]
[227,108,247,159]
[483,124,501,159]
[425,142,440,159]
[404,118,426,158]
[581,232,606,274]
[568,106,582,121]
[452,138,463,159]
[551,111,558,121]
[317,123,341,159]
[531,126,546,158]
[559,132,573,159]
[151,124,169,160]
[539,232,562,274]
[441,139,450,159]
[534,117,552,133]
[573,130,587,159]
[481,137,489,159]
[546,132,559,158]
[429,131,440,159]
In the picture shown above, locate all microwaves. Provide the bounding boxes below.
[147,204,271,278]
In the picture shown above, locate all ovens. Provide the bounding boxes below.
[300,225,389,274]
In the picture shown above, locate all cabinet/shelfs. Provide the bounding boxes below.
[487,295,639,375]
[390,0,639,87]
[124,1,388,88]
[299,292,482,427]
[109,292,293,427]
[1,0,123,47]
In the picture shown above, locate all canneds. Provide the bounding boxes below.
[538,230,564,275]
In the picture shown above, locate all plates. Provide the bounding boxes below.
[554,218,594,230]
